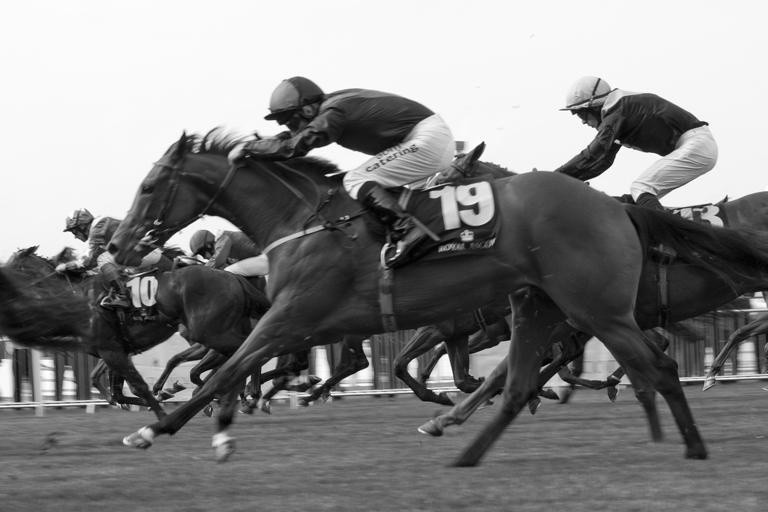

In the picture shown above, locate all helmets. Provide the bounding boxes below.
[63,208,93,232]
[190,230,215,257]
[559,77,618,110]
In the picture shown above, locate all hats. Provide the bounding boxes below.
[264,77,324,125]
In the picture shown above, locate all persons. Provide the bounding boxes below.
[190,230,270,277]
[553,74,718,257]
[55,207,178,306]
[227,76,454,268]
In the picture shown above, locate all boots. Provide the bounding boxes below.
[100,263,132,308]
[636,192,678,258]
[357,181,427,267]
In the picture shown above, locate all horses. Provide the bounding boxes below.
[0,121,768,470]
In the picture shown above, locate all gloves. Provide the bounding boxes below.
[228,142,249,167]
[55,264,66,275]
